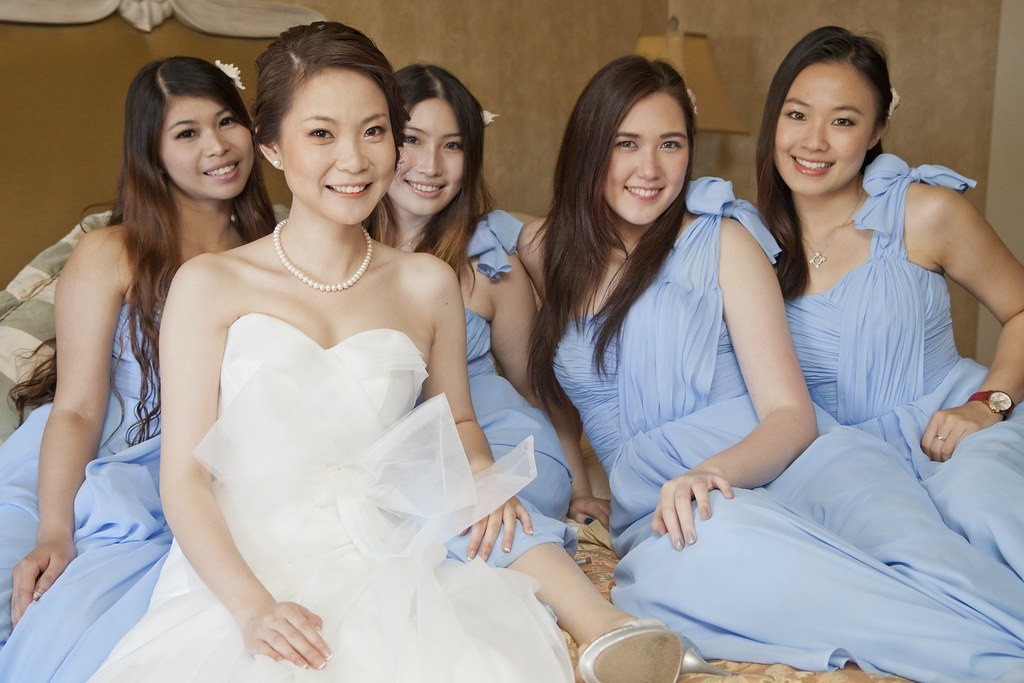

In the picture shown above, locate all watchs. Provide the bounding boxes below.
[969,390,1015,421]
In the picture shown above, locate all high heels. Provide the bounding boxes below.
[577,618,732,683]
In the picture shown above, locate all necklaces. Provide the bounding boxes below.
[408,237,417,250]
[801,189,863,269]
[273,217,373,293]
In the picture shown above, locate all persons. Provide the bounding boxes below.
[0,57,279,683]
[88,21,579,682]
[359,63,738,683]
[756,26,1024,593]
[486,56,1024,683]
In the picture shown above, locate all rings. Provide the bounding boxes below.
[936,435,945,441]
